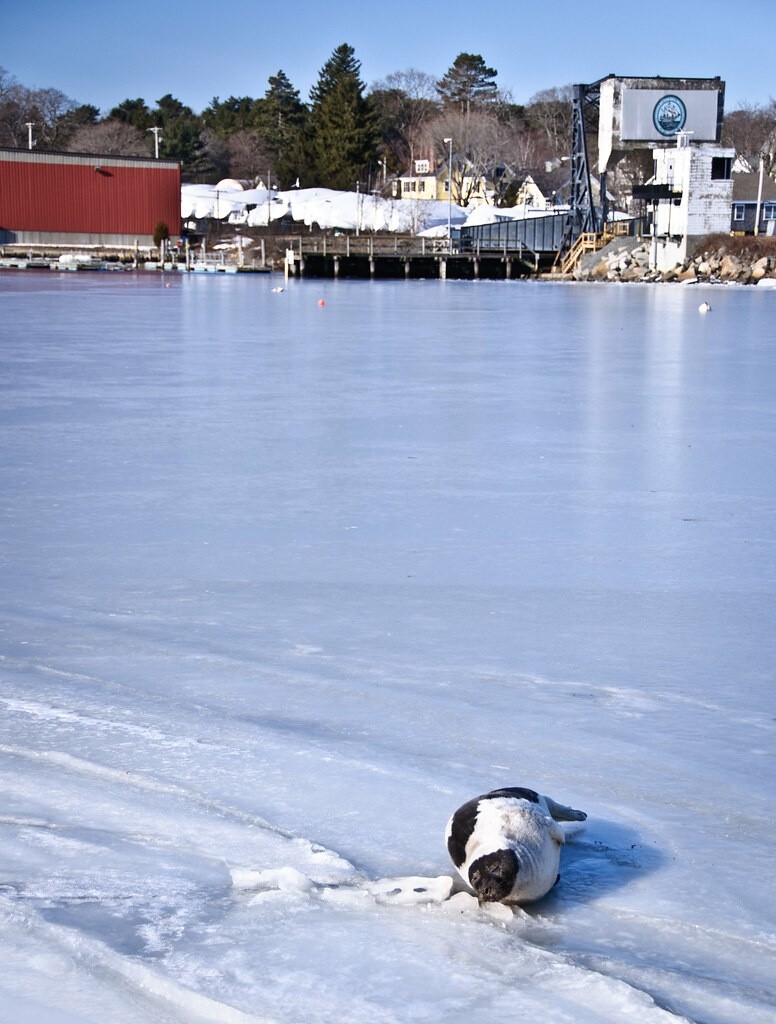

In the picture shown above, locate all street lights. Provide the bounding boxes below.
[444,137,453,239]
